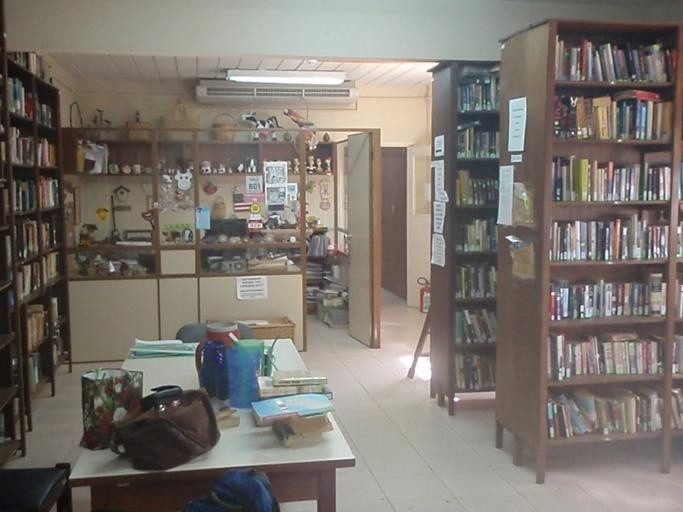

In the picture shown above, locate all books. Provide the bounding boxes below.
[251,368,336,448]
[452,67,501,393]
[0,50,67,421]
[210,396,240,430]
[545,31,683,437]
[130,338,198,359]
[305,225,349,313]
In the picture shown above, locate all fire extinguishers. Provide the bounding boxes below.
[417,277,432,314]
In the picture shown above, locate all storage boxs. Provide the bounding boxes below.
[238,316,296,344]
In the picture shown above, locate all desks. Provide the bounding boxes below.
[68,339,354,512]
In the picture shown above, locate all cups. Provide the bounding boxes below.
[195,323,242,370]
[132,164,144,175]
[225,340,273,409]
[144,167,151,174]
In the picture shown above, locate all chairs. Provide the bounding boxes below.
[0,461,72,512]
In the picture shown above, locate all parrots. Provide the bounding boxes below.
[283,106,314,129]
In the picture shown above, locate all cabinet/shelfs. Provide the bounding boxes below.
[63,127,336,177]
[493,20,682,485]
[426,60,496,417]
[0,59,72,470]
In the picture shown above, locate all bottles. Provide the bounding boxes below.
[196,340,227,402]
[200,160,211,175]
[75,138,84,173]
[212,158,256,174]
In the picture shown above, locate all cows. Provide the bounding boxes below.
[240,111,283,142]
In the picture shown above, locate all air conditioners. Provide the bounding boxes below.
[195,78,356,107]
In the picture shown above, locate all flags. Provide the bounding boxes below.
[233,193,265,212]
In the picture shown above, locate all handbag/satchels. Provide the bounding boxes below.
[79,368,143,450]
[108,385,220,470]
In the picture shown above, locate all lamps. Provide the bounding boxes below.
[224,68,344,87]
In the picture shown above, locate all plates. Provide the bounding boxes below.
[108,163,132,175]
